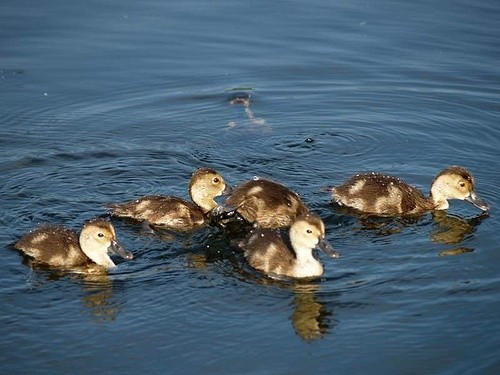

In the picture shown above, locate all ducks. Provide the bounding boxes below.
[104,167,235,231]
[328,166,490,219]
[12,217,134,277]
[209,176,311,232]
[228,213,341,280]
